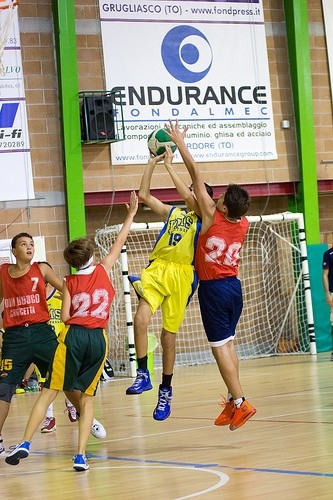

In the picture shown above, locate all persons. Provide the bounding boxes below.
[161,117,257,431]
[0,190,139,471]
[125,144,214,421]
[322,233,333,361]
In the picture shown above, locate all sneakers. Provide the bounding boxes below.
[5,441,30,466]
[125,369,153,395]
[72,454,90,470]
[101,358,114,382]
[64,399,77,422]
[41,417,56,433]
[76,411,106,439]
[153,384,173,422]
[38,383,42,391]
[0,439,5,456]
[18,379,28,389]
[214,393,257,431]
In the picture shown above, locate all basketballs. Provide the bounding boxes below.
[148,130,179,159]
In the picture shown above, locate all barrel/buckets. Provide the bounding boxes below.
[125,332,159,376]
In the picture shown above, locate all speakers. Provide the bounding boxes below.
[82,96,115,140]
